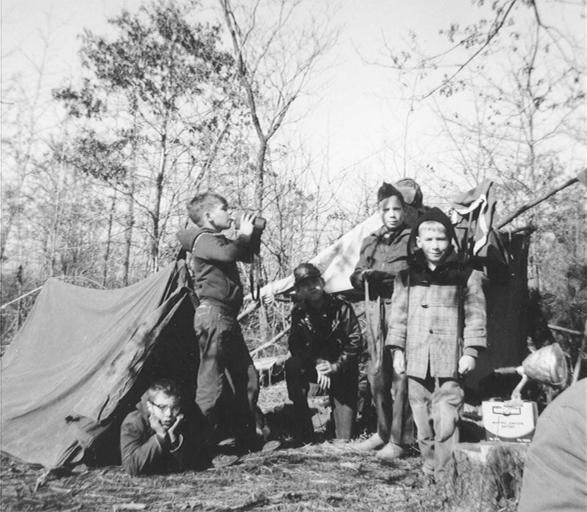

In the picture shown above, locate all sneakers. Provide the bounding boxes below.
[200,453,238,467]
[377,443,404,462]
[355,433,384,451]
[252,439,281,452]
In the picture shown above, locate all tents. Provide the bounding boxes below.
[2,262,201,473]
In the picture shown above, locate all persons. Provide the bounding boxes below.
[175,191,261,467]
[121,378,209,480]
[392,178,431,221]
[517,376,587,511]
[349,181,414,462]
[285,262,363,445]
[385,208,490,487]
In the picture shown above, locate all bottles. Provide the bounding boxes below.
[229,215,267,229]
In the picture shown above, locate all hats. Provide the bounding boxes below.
[377,183,399,204]
[291,263,319,284]
